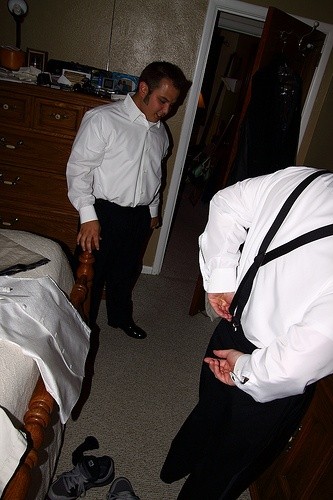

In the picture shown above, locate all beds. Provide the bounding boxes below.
[0,228,96,500]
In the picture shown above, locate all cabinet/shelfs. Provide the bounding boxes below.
[249,373,333,500]
[0,77,112,262]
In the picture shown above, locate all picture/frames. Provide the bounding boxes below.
[54,61,140,98]
[25,48,49,73]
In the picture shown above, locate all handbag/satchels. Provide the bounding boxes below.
[187,143,217,190]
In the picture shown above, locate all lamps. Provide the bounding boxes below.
[0,0,30,70]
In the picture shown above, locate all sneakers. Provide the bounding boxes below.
[106,476,140,500]
[48,454,116,500]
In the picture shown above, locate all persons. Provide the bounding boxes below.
[158,165,333,500]
[66,59,193,338]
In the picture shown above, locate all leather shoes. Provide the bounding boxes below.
[108,319,147,339]
